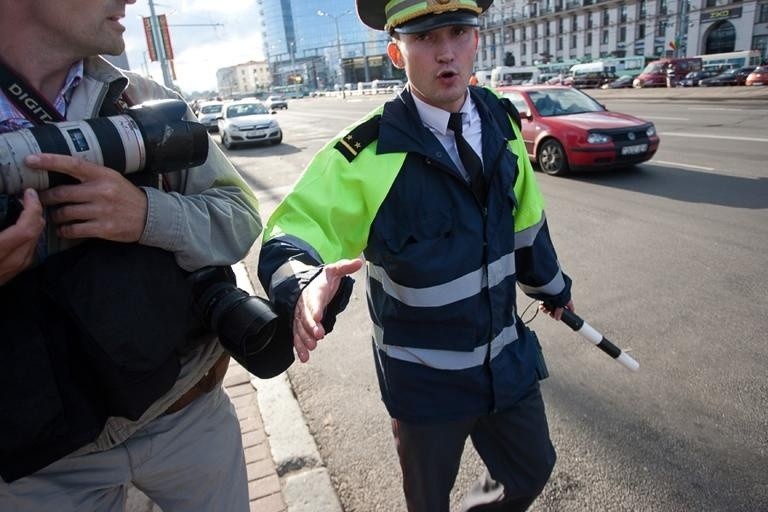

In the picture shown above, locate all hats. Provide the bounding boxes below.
[355,0,494,35]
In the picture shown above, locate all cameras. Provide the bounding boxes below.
[176,265,295,379]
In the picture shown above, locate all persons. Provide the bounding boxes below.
[257,0,576,511]
[468,71,478,85]
[665,63,675,88]
[0,1,264,511]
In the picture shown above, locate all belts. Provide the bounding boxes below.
[160,345,231,420]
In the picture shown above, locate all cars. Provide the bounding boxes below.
[484,82,659,176]
[197,94,290,147]
[472,47,768,89]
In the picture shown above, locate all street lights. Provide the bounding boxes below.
[317,6,354,90]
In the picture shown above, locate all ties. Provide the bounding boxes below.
[449,111,486,212]
